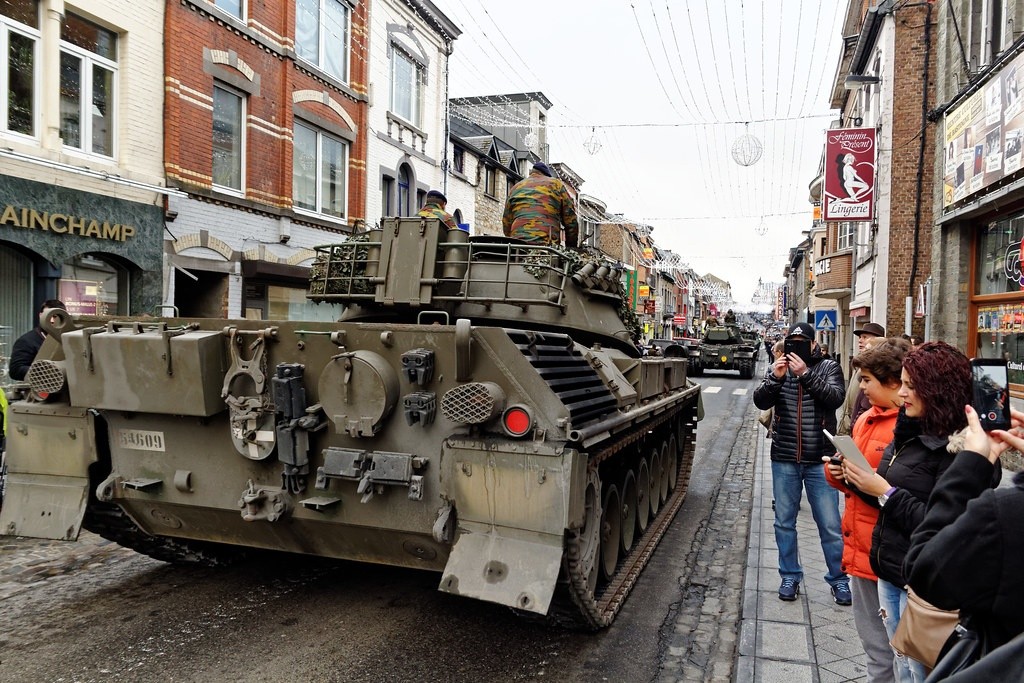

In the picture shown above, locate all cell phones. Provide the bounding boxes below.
[970,356,1012,431]
[784,339,812,368]
[830,456,843,466]
[823,429,875,474]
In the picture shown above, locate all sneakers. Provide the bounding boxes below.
[831,583,852,606]
[778,577,800,600]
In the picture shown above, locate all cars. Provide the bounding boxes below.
[648,339,678,351]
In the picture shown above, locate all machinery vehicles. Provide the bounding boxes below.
[974,380,1001,412]
[0,215,701,631]
[686,321,757,379]
[741,329,761,361]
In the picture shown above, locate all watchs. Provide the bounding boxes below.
[878,487,896,506]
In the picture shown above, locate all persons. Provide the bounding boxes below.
[981,375,1007,422]
[842,341,1002,683]
[821,344,833,360]
[9,299,67,381]
[749,311,784,363]
[413,190,458,228]
[503,162,579,250]
[724,309,736,324]
[753,323,852,605]
[825,337,912,683]
[901,404,1024,664]
[837,325,887,437]
[978,316,984,329]
[742,323,751,330]
[704,311,720,331]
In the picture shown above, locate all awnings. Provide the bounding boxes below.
[688,326,694,334]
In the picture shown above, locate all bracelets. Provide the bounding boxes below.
[801,368,811,378]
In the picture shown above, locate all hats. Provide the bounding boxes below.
[853,323,884,337]
[533,162,552,177]
[785,322,815,342]
[427,191,447,205]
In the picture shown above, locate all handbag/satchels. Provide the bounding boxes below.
[758,408,772,429]
[889,584,961,669]
